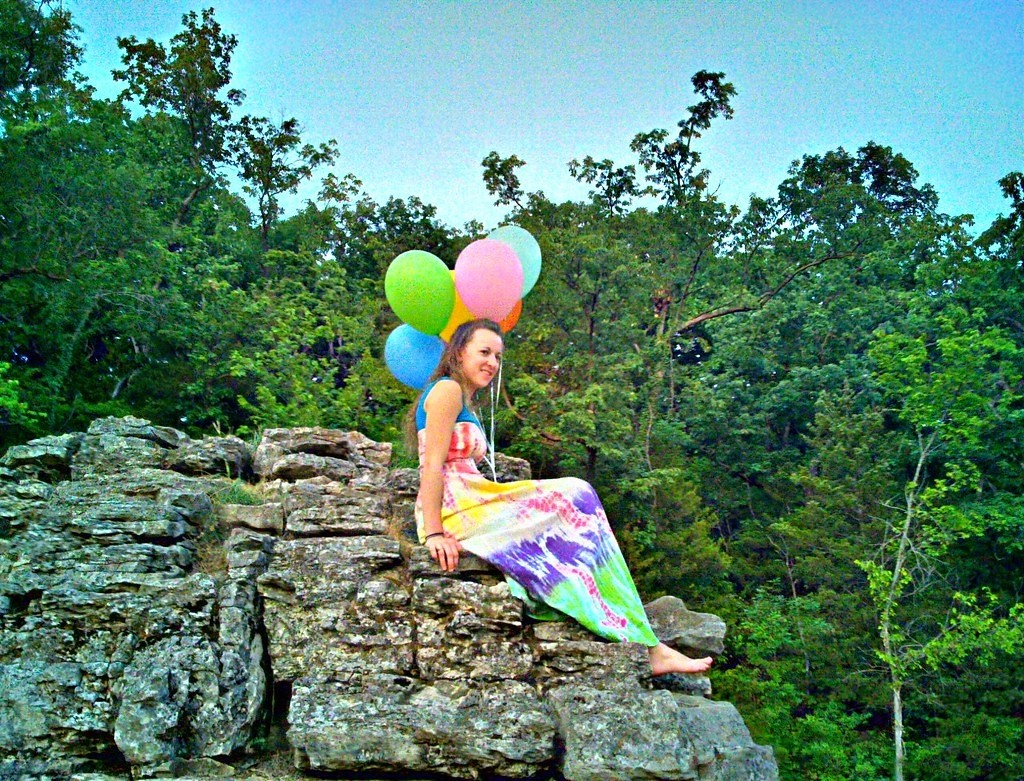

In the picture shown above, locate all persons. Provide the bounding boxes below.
[405,318,712,676]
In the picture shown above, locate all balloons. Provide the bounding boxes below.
[385,250,455,335]
[454,239,524,322]
[385,324,446,390]
[487,225,542,300]
[439,270,476,344]
[498,299,522,333]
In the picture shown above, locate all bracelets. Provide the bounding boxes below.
[426,532,444,539]
